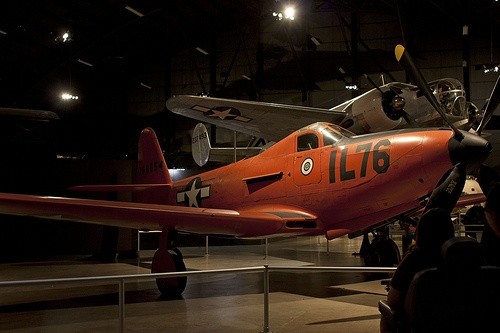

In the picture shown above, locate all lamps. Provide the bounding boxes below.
[58,90,79,103]
[50,27,73,43]
[272,4,299,25]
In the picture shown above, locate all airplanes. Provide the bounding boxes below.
[0,44,500,291]
[165,75,500,166]
